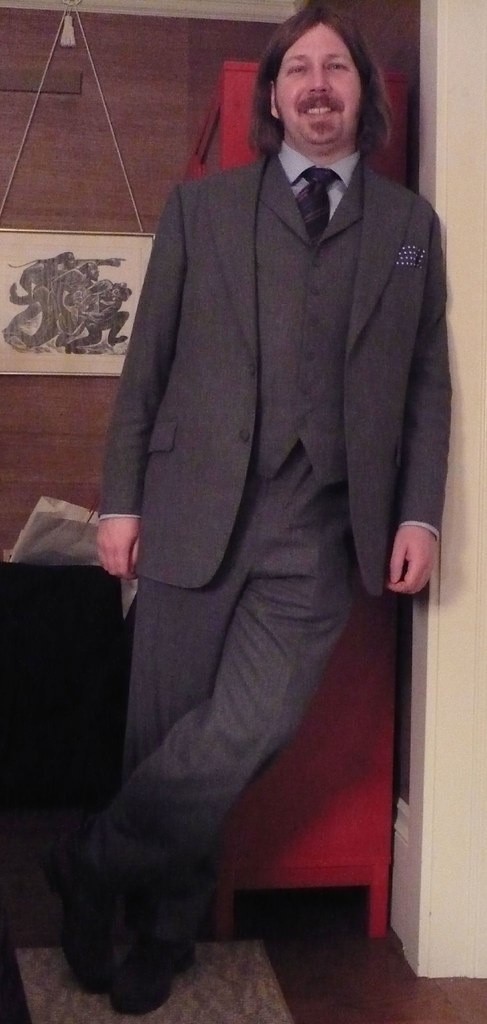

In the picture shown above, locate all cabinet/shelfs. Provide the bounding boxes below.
[189,57,411,942]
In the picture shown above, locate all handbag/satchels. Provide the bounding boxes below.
[10,494,139,620]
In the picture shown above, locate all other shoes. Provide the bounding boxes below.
[107,899,196,1016]
[46,837,119,994]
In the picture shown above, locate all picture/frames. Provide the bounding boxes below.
[0,227,155,377]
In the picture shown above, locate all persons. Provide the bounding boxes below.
[45,6,451,1015]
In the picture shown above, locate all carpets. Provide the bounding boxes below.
[14,941,297,1024]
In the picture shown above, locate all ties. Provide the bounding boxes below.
[295,167,342,243]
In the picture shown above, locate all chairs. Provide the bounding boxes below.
[1,562,123,817]
